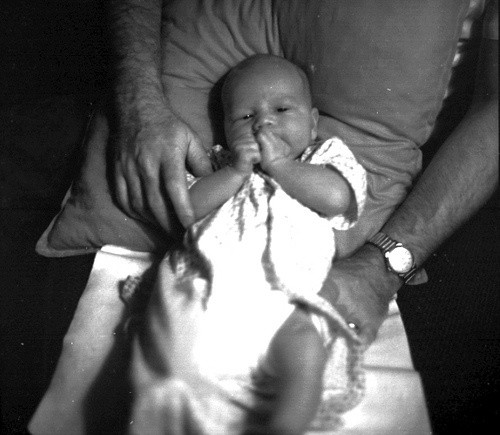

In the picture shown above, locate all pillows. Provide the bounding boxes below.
[33,1,482,259]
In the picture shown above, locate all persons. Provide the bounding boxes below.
[30,0,500,435]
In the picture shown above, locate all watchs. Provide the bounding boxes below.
[368,228,422,285]
